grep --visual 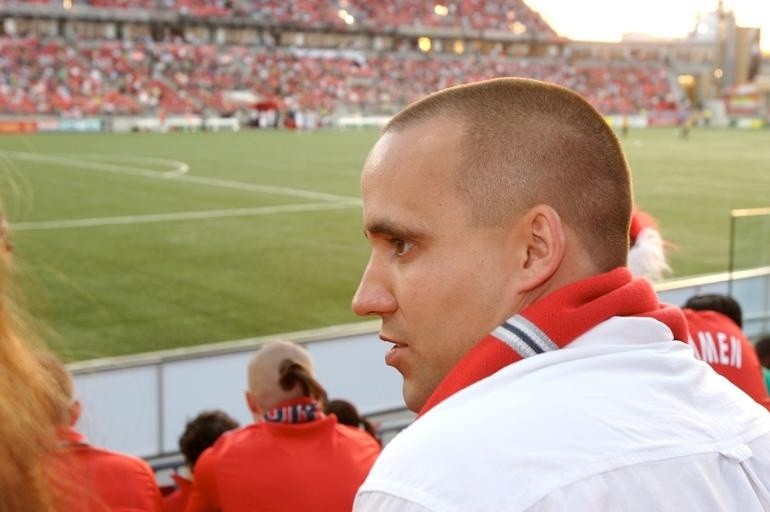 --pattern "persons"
[326,398,382,448]
[1,1,709,137]
[679,291,769,408]
[350,74,770,511]
[164,408,240,512]
[185,339,382,512]
[29,354,165,511]
[755,336,770,394]
